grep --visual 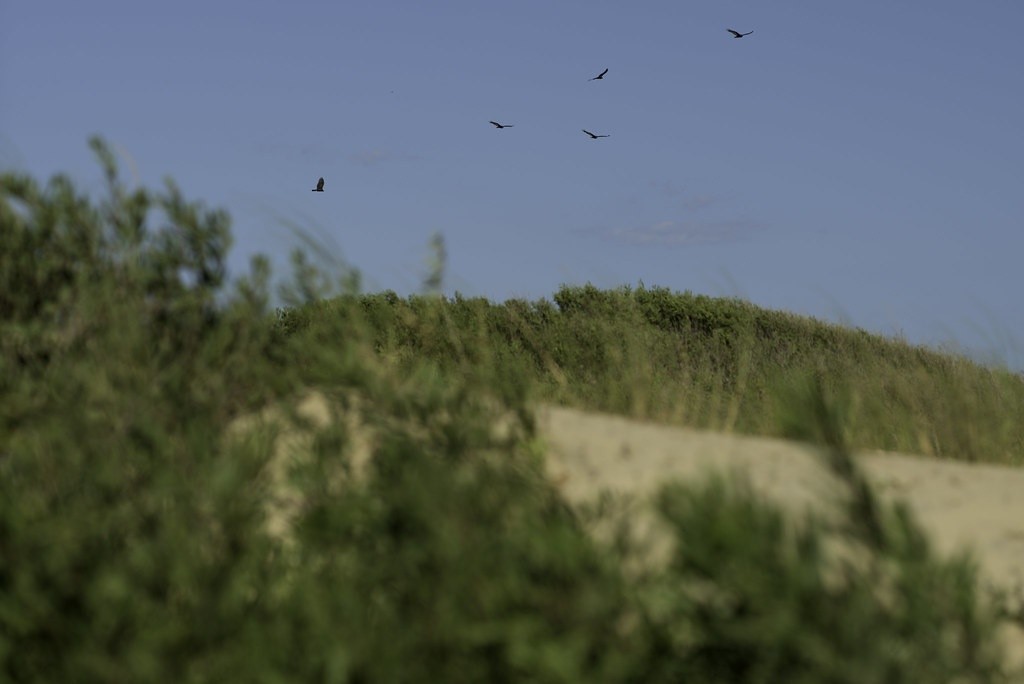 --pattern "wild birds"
[488,121,514,129]
[311,176,325,192]
[582,130,610,139]
[726,27,754,38]
[587,67,609,81]
